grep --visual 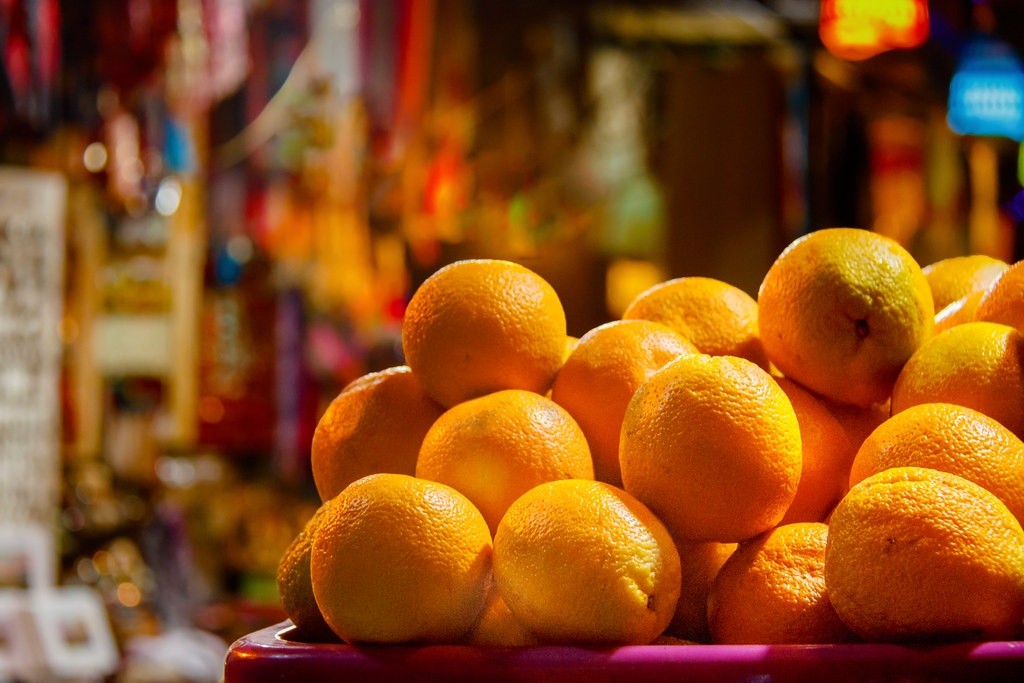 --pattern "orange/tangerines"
[277,226,1023,650]
